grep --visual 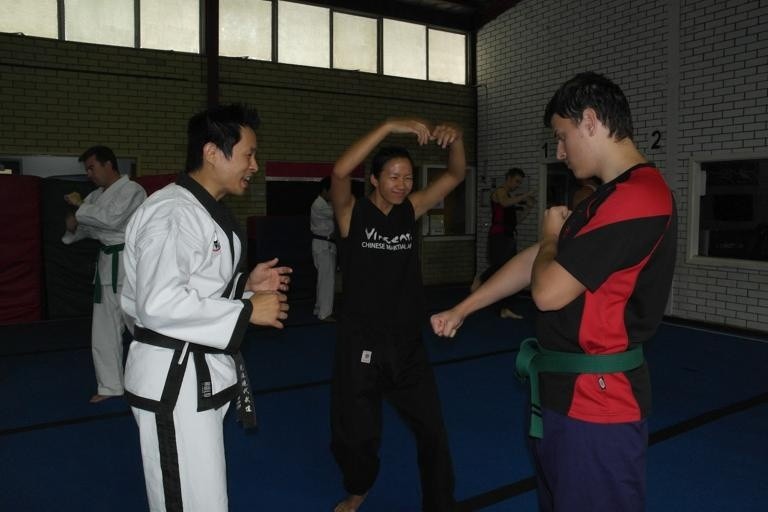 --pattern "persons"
[61,145,148,404]
[121,102,294,512]
[470,168,537,320]
[430,71,678,512]
[309,176,337,323]
[329,117,466,512]
